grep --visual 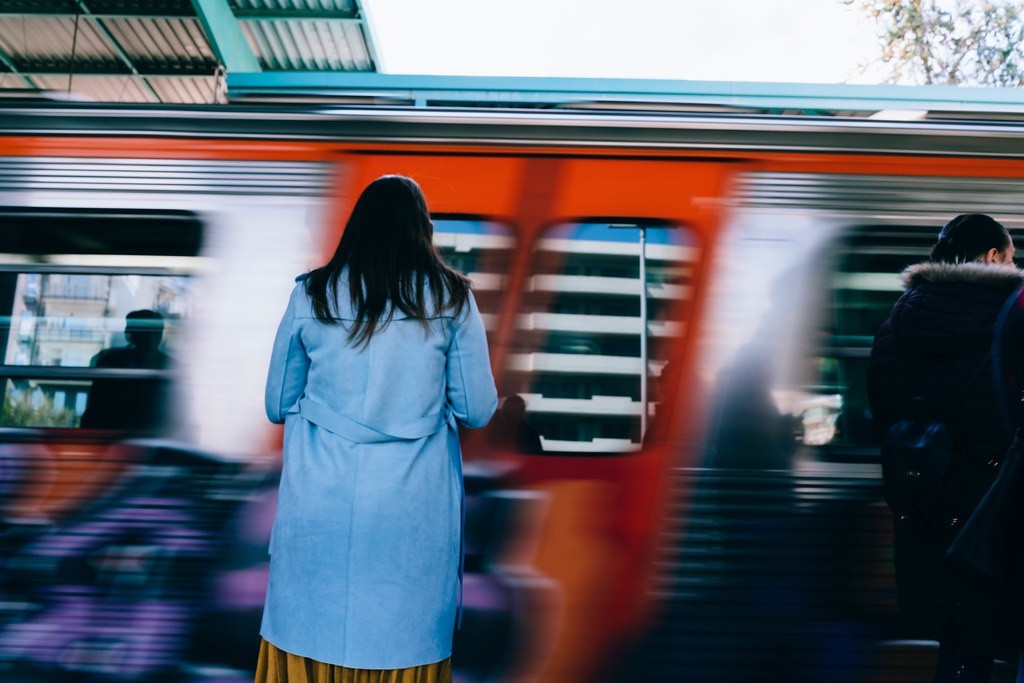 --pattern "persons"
[79,307,180,433]
[254,174,500,683]
[870,211,1024,681]
